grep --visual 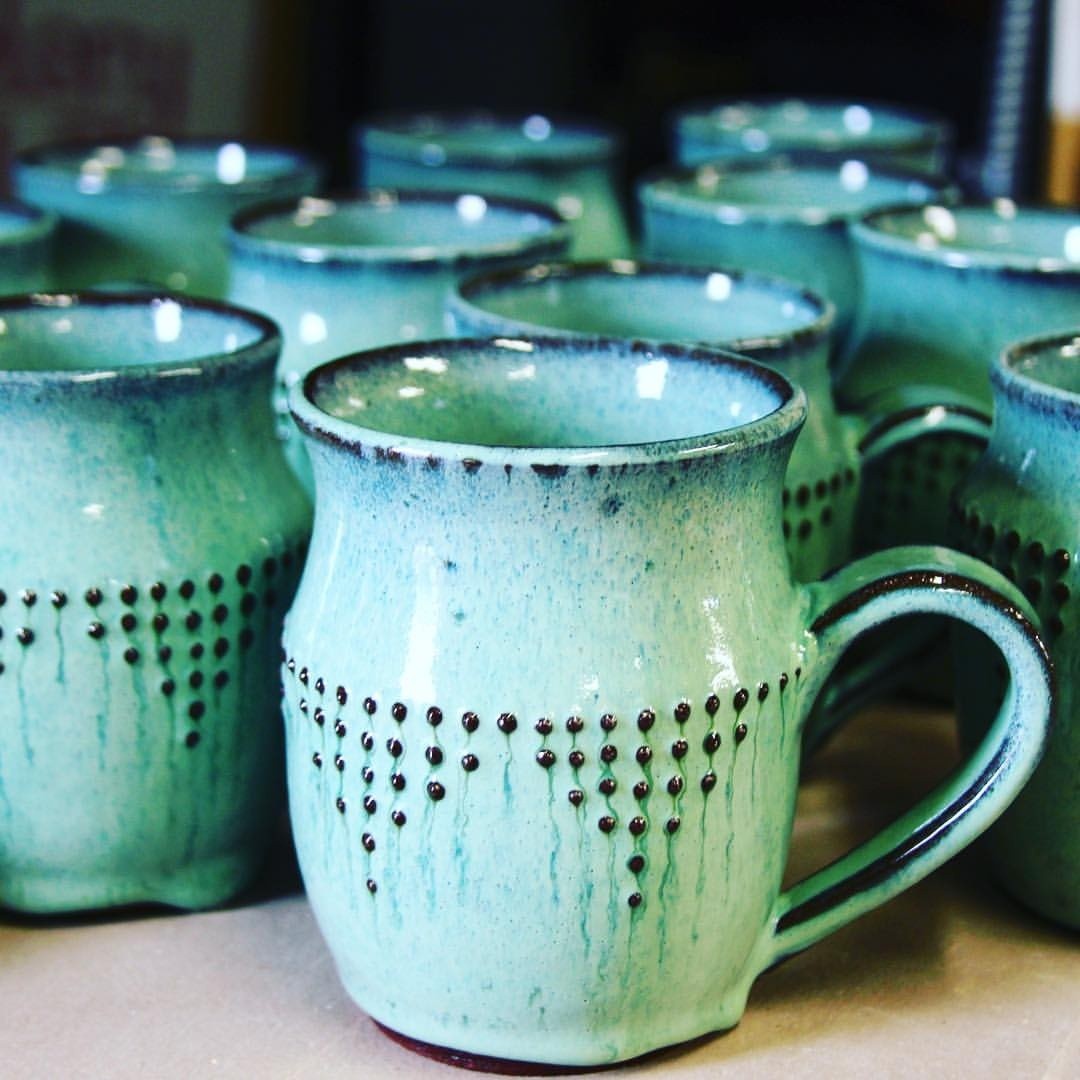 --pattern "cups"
[280,332,1058,1069]
[0,290,318,908]
[0,98,1080,771]
[947,329,1079,928]
